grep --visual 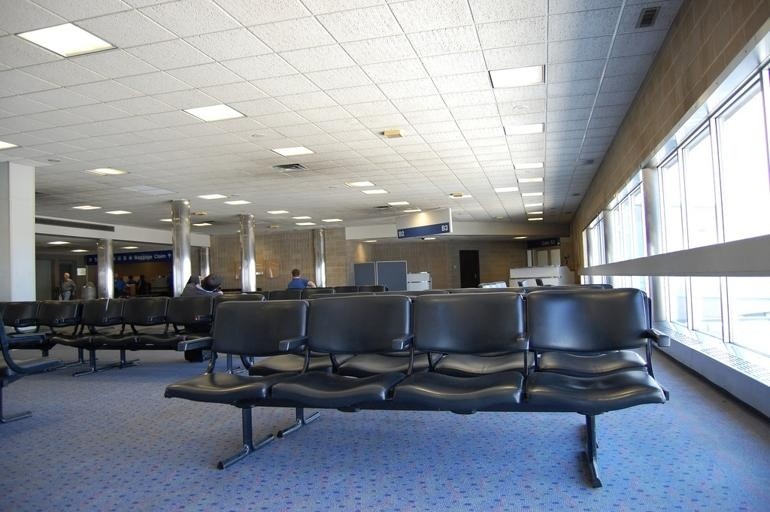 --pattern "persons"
[180,273,223,333]
[58,272,76,301]
[288,269,316,289]
[116,273,146,297]
